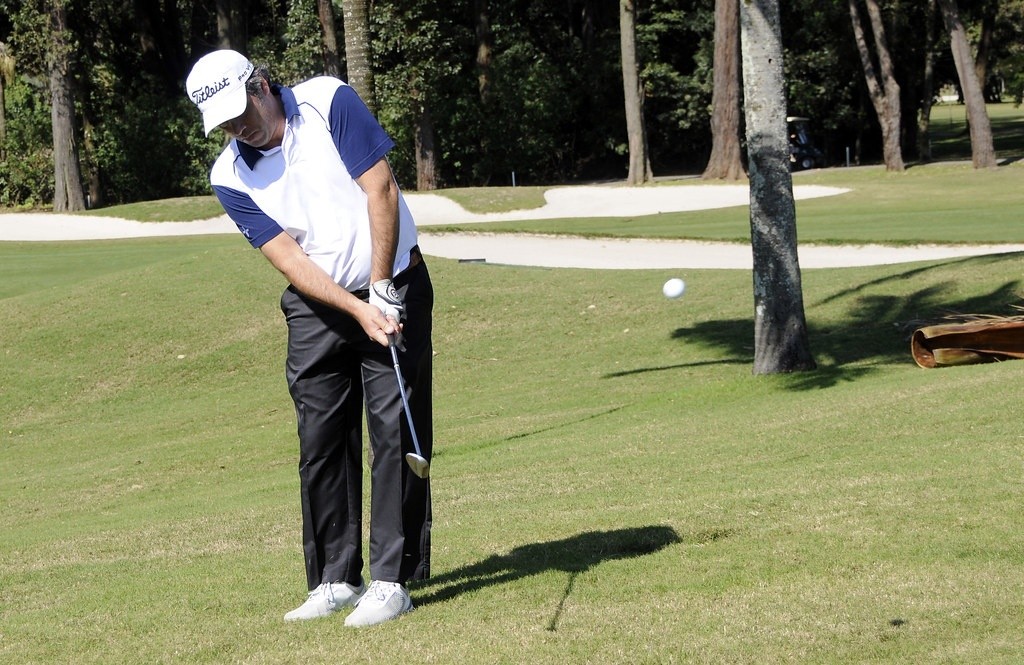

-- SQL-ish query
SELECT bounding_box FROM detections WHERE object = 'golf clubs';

[385,331,429,479]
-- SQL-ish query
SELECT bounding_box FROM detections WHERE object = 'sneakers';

[346,578,413,627]
[284,579,365,620]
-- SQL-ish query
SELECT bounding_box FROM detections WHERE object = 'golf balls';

[662,278,685,298]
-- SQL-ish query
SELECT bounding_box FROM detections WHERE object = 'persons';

[186,50,433,627]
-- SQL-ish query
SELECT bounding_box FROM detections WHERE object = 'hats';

[187,48,256,137]
